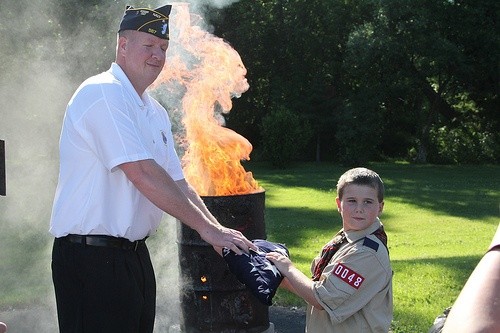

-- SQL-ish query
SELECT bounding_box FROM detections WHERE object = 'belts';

[58,234,145,253]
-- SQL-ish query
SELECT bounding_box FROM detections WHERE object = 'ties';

[311,225,389,281]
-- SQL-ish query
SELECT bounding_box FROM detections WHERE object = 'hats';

[117,5,172,40]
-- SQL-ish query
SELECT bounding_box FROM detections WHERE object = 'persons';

[264,168,394,333]
[442,222,500,333]
[50,4,258,333]
